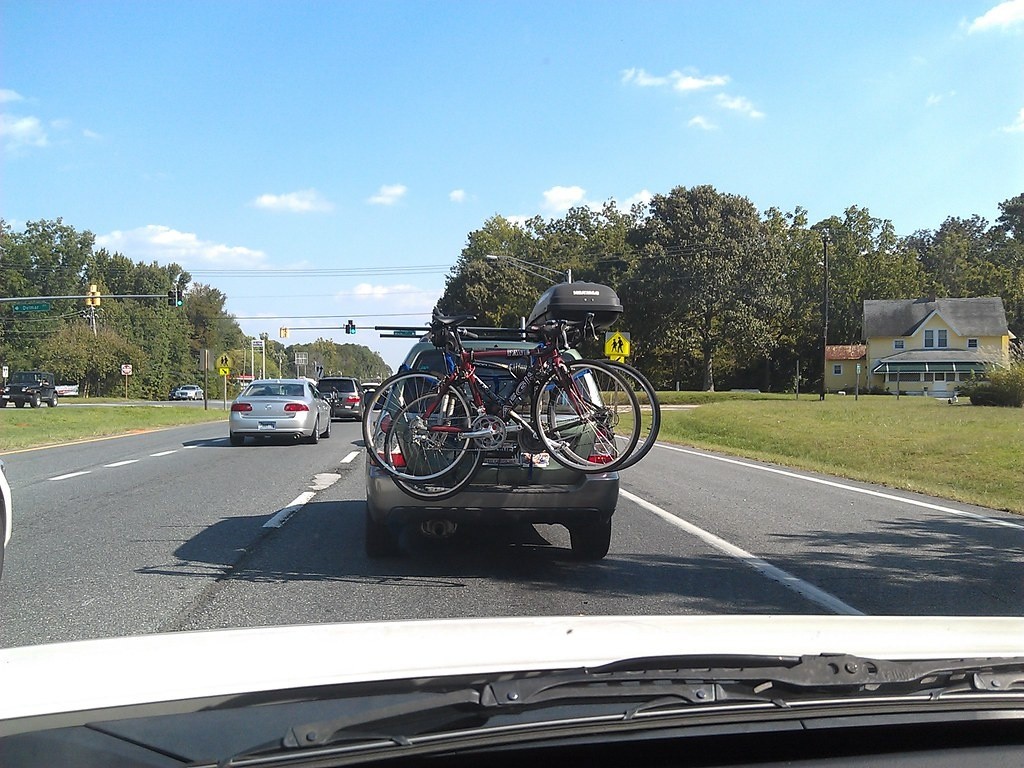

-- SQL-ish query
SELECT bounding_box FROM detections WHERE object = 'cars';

[168,385,204,400]
[360,382,387,411]
[230,379,333,447]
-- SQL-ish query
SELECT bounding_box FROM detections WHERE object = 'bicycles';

[359,309,663,502]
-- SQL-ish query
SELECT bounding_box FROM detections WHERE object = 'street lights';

[486,255,572,284]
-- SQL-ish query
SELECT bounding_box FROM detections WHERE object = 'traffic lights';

[177,290,183,306]
[351,325,355,334]
[346,325,350,334]
[347,320,353,324]
[169,290,176,305]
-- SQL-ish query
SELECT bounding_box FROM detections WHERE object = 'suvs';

[316,377,366,420]
[368,326,620,562]
[0,371,58,409]
